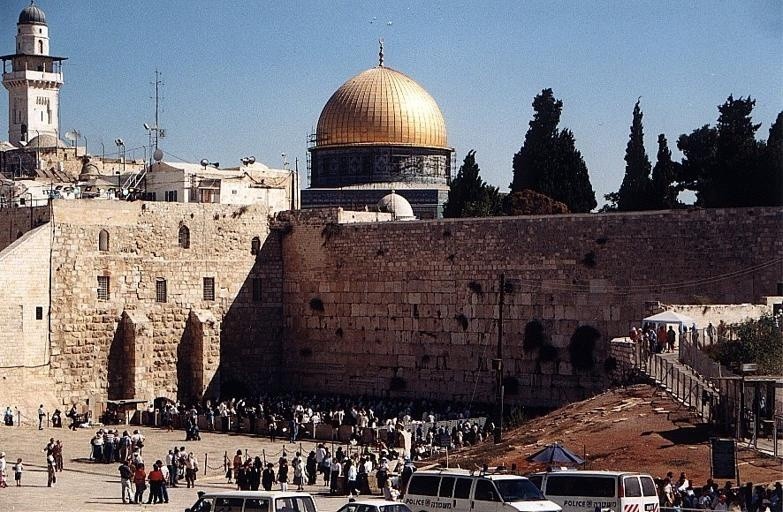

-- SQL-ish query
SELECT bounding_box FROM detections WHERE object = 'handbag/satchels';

[52,471,57,484]
[193,463,199,472]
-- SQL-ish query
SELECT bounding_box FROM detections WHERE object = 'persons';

[651,472,782,512]
[751,392,767,431]
[629,317,729,364]
[1,390,496,512]
[33,215,42,227]
[15,226,23,239]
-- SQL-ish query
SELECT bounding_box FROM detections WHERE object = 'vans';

[185,489,317,511]
[527,470,661,511]
[403,468,563,512]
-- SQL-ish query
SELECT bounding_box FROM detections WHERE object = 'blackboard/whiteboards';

[710,438,737,480]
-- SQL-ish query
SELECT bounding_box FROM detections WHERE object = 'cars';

[334,500,413,511]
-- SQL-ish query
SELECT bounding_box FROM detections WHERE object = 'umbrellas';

[522,441,586,470]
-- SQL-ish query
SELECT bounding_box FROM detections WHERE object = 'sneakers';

[296,487,304,490]
[345,495,354,498]
[156,501,170,504]
[143,500,156,504]
[122,500,133,504]
[355,490,361,498]
[134,501,143,504]
[186,484,195,488]
